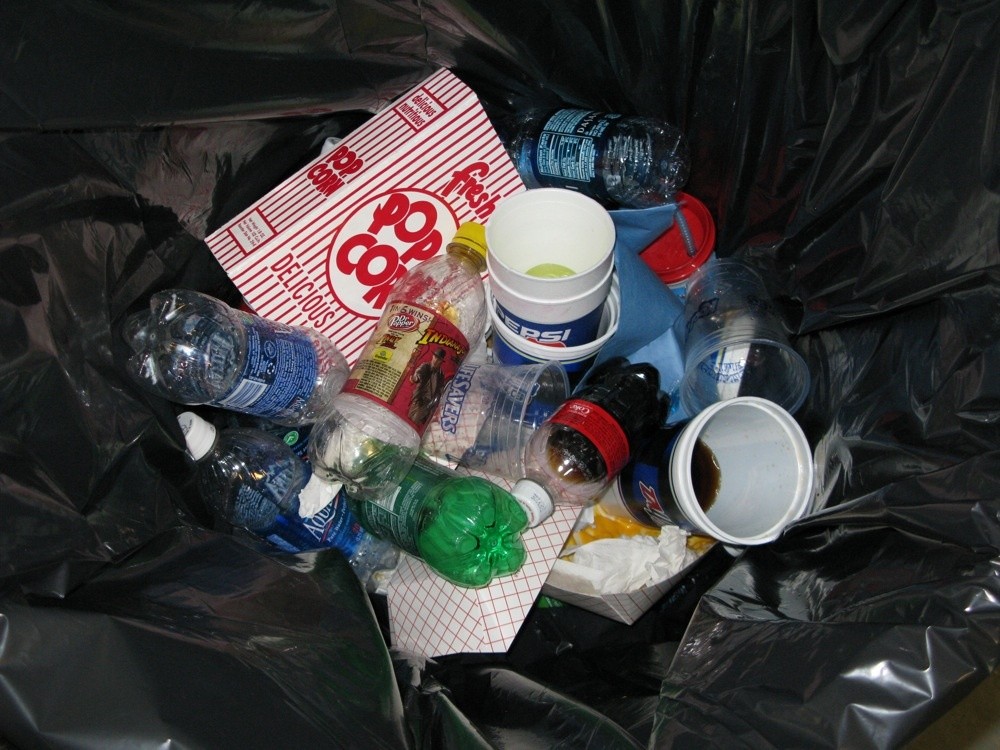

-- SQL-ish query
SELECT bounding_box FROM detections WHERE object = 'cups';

[598,396,815,546]
[420,359,571,481]
[678,258,813,418]
[484,188,623,428]
[634,190,718,306]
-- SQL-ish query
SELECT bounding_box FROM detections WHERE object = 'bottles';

[497,102,692,211]
[350,453,529,589]
[307,221,488,503]
[509,355,672,529]
[176,411,401,594]
[118,287,350,428]
